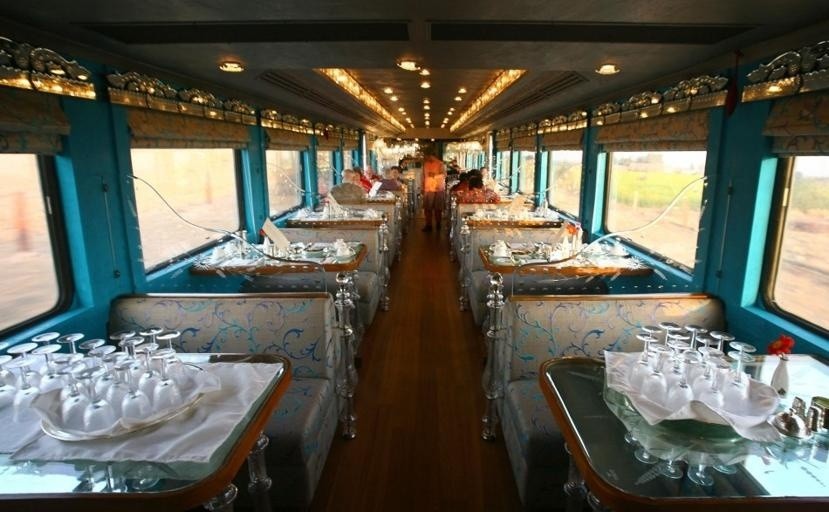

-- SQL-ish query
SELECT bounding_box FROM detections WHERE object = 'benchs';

[107,177,414,507]
[452,191,727,505]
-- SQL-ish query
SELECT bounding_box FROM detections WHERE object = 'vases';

[770,357,790,394]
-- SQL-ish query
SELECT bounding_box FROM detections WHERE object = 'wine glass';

[0,327,198,434]
[624,431,739,487]
[76,461,161,494]
[629,320,758,415]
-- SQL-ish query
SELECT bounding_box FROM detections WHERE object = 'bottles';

[262,236,271,260]
[770,355,790,400]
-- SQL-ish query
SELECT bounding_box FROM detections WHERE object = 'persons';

[341,159,408,194]
[449,159,500,203]
[415,147,447,233]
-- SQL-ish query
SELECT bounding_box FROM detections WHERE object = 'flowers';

[768,335,796,359]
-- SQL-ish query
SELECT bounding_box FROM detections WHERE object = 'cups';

[334,238,350,257]
[489,239,507,257]
